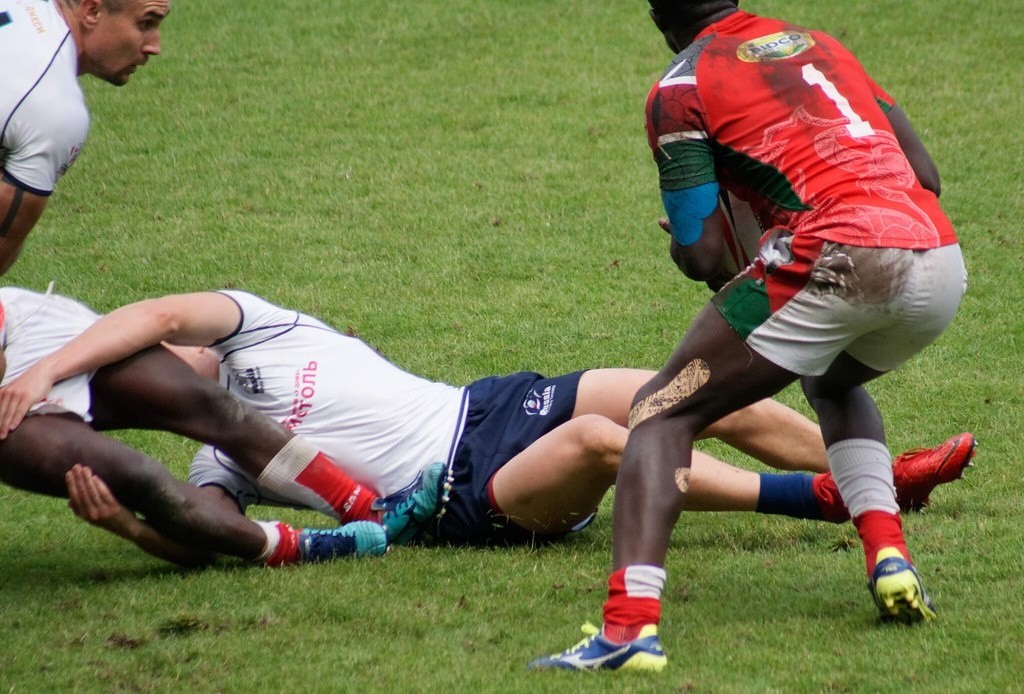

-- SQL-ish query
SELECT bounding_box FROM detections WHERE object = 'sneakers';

[894,434,978,512]
[527,621,668,671]
[868,546,938,623]
[298,520,389,564]
[814,473,852,524]
[371,462,454,546]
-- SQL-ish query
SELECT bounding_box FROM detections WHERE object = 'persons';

[0,0,171,276]
[533,0,967,672]
[1,288,978,567]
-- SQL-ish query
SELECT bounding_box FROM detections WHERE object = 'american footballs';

[712,187,767,276]
